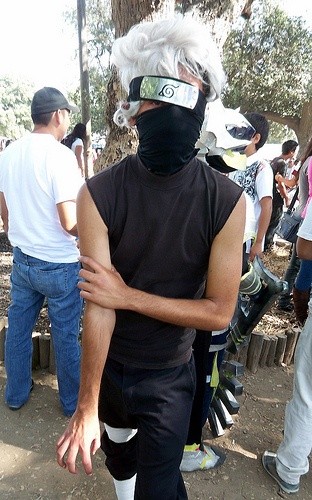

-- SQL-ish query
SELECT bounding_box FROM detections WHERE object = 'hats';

[31,86,78,117]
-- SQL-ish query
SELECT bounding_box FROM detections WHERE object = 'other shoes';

[9,379,34,410]
[278,304,294,310]
[180,444,226,472]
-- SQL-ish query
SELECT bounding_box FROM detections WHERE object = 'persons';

[0,91,312,494]
[54,16,247,500]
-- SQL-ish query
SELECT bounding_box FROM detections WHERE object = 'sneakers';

[262,449,300,493]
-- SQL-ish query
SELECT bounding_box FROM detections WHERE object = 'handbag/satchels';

[276,213,300,243]
